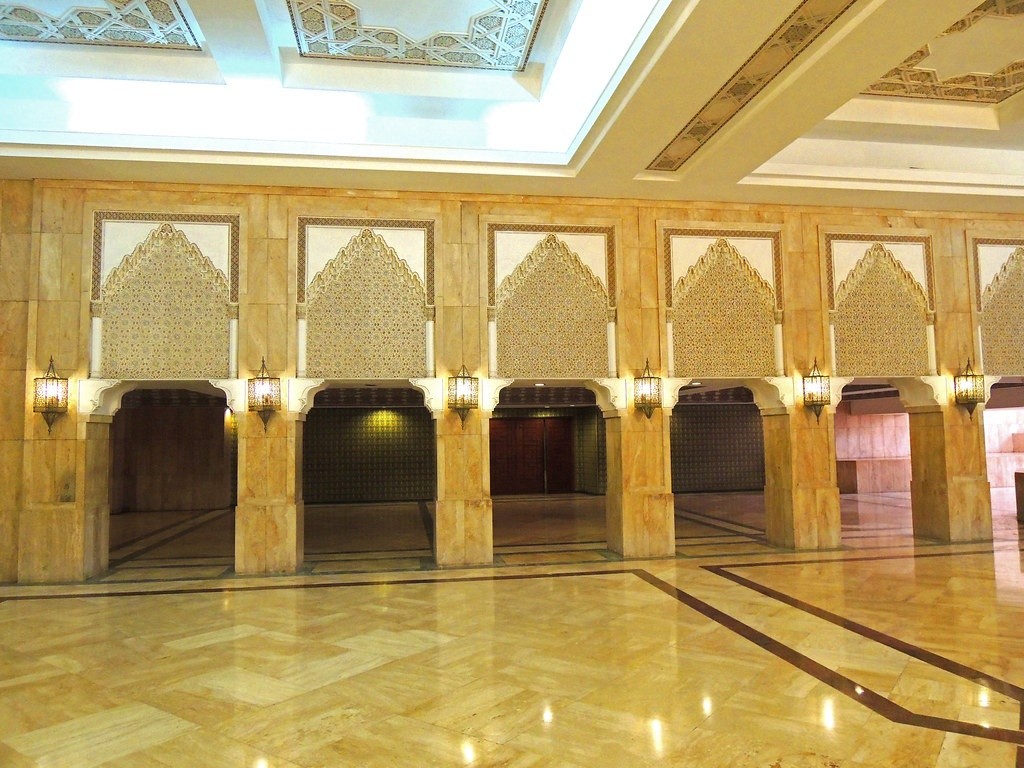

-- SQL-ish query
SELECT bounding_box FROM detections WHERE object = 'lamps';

[448,365,478,430]
[954,357,985,421]
[248,355,281,433]
[634,358,663,419]
[803,357,830,425]
[33,355,68,436]
